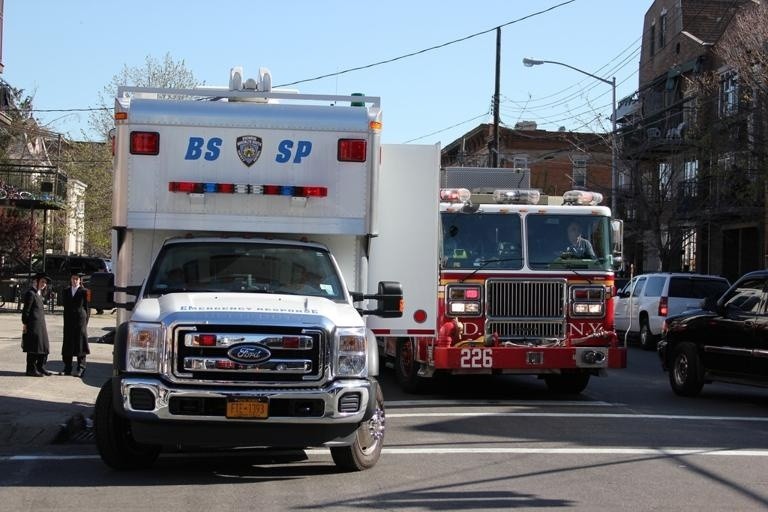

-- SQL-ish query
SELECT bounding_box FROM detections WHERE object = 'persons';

[277,263,321,295]
[59,274,92,378]
[443,225,459,270]
[553,221,604,269]
[21,272,53,376]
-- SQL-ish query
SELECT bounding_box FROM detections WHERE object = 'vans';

[615,272,733,347]
[0,253,112,304]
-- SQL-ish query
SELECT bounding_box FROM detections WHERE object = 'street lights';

[523,55,618,218]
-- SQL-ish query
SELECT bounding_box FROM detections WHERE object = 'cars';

[655,268,767,396]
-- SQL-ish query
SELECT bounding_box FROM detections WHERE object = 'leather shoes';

[59,366,72,375]
[26,369,42,376]
[37,367,49,375]
[74,366,85,377]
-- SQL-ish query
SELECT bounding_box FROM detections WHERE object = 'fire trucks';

[379,167,625,392]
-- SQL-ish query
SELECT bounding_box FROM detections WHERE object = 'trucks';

[92,67,402,471]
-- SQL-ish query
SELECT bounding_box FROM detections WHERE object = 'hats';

[32,271,52,285]
[71,269,86,277]
